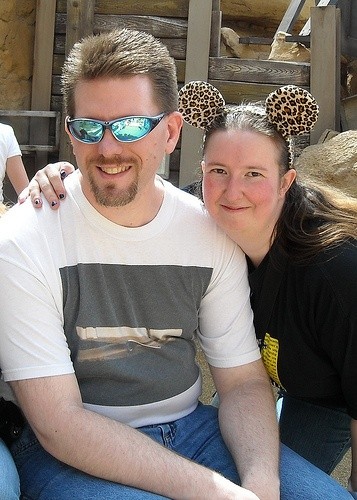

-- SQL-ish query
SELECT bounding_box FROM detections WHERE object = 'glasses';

[65,109,171,145]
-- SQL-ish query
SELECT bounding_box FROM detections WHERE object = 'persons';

[0,123,31,214]
[0,436,22,500]
[0,29,355,500]
[17,109,357,495]
